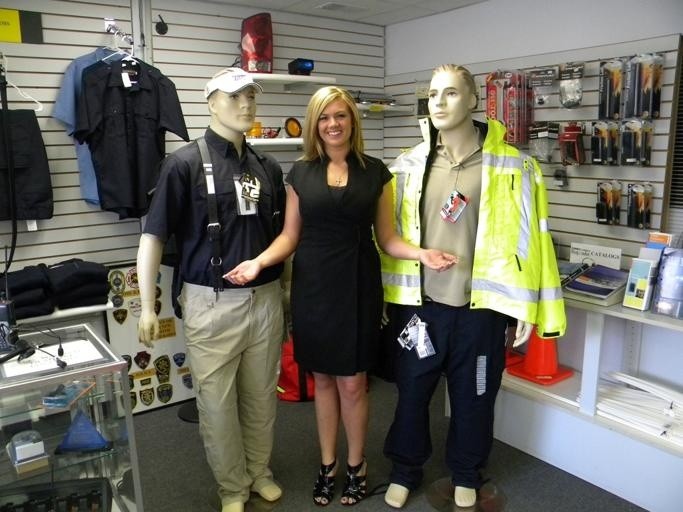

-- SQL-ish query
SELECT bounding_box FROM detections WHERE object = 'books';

[557,259,629,306]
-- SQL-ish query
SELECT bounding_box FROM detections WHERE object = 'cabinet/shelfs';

[245,73,416,146]
[1,323,142,512]
[445,260,683,511]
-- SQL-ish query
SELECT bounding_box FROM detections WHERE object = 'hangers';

[99,26,142,82]
[0,58,45,113]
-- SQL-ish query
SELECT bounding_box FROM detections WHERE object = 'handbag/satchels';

[277,338,369,401]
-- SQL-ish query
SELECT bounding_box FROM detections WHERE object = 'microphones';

[14,321,64,357]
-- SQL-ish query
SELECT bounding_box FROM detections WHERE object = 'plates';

[285,117,302,137]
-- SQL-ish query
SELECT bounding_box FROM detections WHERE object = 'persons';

[222,86,457,505]
[137,68,286,511]
[385,63,566,508]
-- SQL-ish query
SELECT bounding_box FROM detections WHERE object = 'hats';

[204,67,264,99]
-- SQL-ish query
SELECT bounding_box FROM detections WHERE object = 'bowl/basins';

[261,127,281,138]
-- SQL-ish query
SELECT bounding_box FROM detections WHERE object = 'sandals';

[342,460,367,506]
[313,457,336,507]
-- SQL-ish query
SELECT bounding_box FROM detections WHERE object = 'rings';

[241,283,245,285]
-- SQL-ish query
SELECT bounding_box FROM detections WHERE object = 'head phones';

[0,324,33,365]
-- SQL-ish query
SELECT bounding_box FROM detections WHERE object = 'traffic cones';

[506,316,575,388]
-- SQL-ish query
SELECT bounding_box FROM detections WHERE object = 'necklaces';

[327,167,347,184]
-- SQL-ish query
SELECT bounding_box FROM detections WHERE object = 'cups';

[246,122,261,138]
